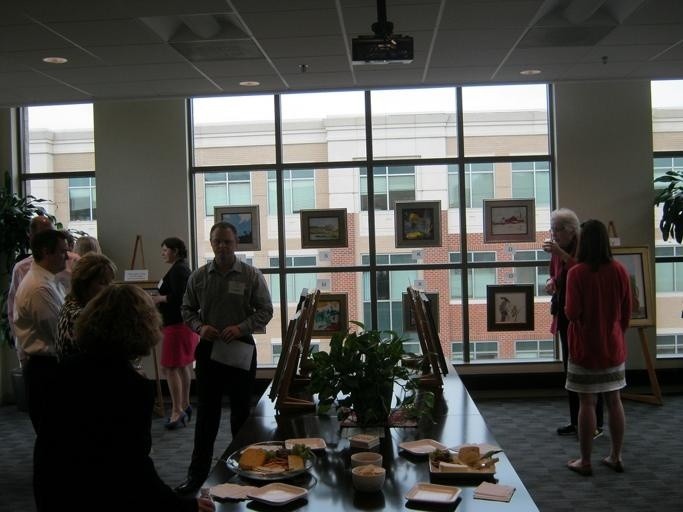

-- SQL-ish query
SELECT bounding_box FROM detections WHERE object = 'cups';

[543,238,553,252]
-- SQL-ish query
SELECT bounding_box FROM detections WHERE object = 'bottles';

[199,488,213,502]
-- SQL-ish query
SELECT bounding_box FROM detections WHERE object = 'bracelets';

[197,324,203,334]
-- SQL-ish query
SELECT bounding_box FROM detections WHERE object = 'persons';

[173,222,273,497]
[31,283,216,512]
[543,207,604,444]
[12,216,148,434]
[564,218,633,476]
[149,236,199,429]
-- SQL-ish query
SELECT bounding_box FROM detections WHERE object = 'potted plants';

[301,321,438,431]
[0,172,89,412]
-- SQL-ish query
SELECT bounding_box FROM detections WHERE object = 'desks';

[252,357,481,414]
[195,414,538,511]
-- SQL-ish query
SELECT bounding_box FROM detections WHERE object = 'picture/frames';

[402,291,440,333]
[304,293,350,338]
[214,204,261,252]
[394,200,442,249]
[483,198,537,244]
[299,208,349,250]
[610,246,655,326]
[486,283,535,332]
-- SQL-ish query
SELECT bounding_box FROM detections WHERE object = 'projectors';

[351,34,415,65]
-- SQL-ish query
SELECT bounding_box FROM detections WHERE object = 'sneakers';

[599,454,624,473]
[592,427,604,439]
[567,458,592,476]
[557,424,578,436]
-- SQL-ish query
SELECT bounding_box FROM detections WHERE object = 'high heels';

[164,406,192,429]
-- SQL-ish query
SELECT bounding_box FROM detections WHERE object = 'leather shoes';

[176,480,196,494]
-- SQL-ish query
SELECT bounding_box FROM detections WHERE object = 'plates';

[284,437,327,451]
[428,451,501,474]
[404,481,463,504]
[397,438,450,457]
[245,481,309,507]
[224,440,318,481]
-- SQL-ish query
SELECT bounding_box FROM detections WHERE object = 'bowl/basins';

[350,451,384,469]
[351,465,387,493]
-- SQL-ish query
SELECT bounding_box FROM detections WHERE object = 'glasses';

[551,227,564,232]
